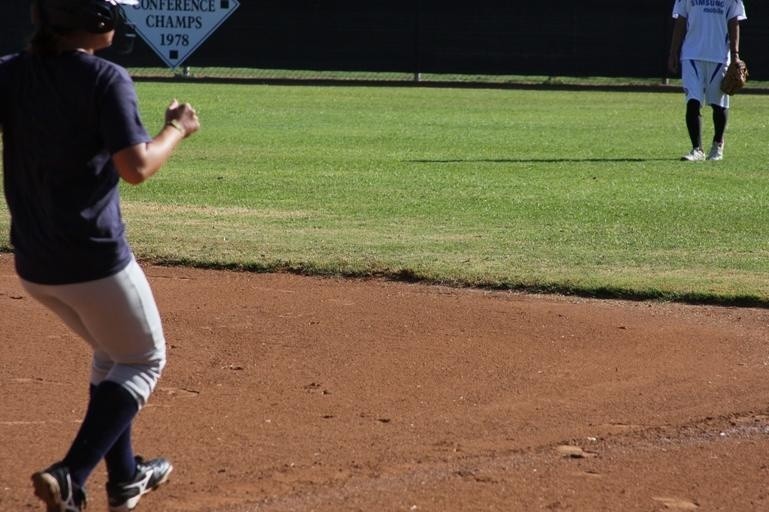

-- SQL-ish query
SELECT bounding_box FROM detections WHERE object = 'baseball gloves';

[720,59,748,96]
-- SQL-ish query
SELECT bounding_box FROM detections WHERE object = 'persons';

[665,1,750,162]
[1,0,204,512]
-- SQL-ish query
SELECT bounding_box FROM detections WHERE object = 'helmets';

[29,0,120,30]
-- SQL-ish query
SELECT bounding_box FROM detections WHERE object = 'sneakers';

[681,147,706,161]
[708,135,724,161]
[106,455,173,512]
[32,462,87,512]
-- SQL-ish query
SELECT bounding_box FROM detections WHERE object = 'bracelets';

[165,117,186,136]
